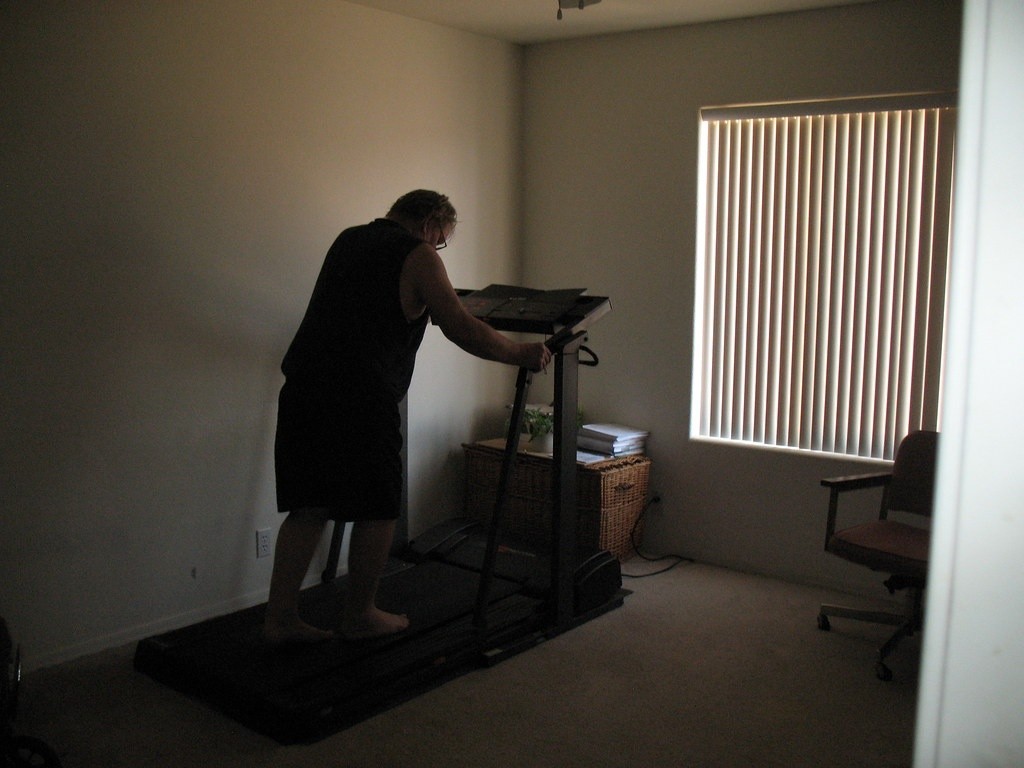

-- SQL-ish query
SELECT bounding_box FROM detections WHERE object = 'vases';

[530,431,554,452]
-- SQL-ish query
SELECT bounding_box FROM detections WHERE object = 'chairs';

[817,430,938,682]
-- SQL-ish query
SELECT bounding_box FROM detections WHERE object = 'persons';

[261,190,552,648]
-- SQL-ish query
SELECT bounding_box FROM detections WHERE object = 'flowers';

[503,397,586,443]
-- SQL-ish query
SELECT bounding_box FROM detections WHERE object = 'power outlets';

[255,528,272,558]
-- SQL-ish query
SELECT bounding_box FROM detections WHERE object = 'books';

[576,422,652,456]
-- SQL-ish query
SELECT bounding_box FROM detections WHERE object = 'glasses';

[435,221,447,250]
